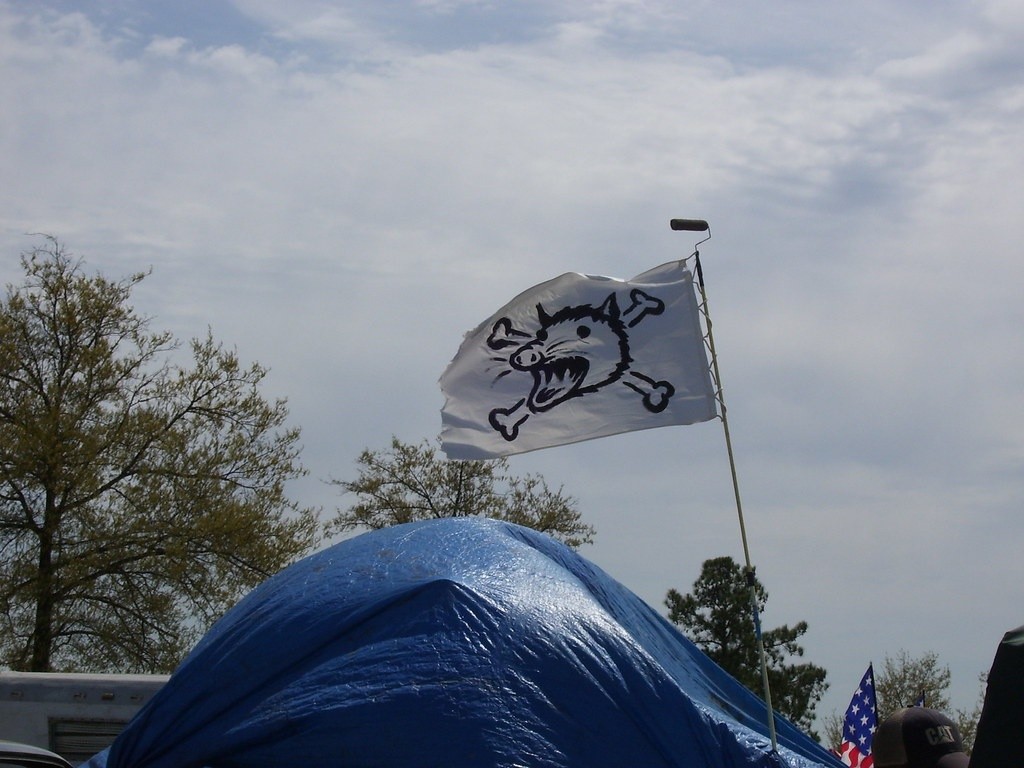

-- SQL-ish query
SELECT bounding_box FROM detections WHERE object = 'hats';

[871,704,964,768]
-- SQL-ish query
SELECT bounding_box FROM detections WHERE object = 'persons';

[871,705,970,768]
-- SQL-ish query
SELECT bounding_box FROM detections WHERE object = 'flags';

[839,662,878,768]
[436,257,718,462]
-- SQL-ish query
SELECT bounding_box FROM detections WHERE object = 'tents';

[80,516,848,768]
